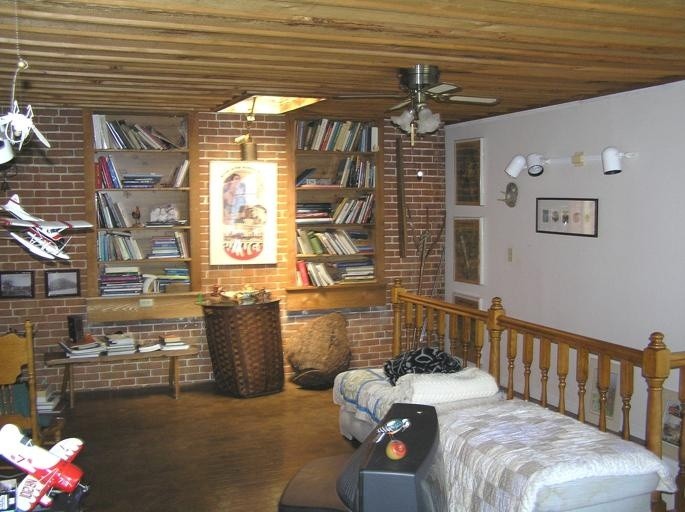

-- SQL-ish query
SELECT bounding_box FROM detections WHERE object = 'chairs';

[0,319,64,470]
[277,405,383,511]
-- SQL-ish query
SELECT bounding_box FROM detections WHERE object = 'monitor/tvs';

[277,402,449,512]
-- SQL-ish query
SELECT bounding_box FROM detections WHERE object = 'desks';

[196,297,285,398]
[16,397,69,414]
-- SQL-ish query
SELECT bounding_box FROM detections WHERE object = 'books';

[90,105,196,297]
[292,115,381,291]
[53,312,192,359]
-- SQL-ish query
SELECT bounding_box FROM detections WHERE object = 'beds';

[331,352,680,511]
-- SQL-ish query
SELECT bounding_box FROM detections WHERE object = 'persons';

[223,172,262,226]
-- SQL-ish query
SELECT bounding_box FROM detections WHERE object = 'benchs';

[43,343,199,408]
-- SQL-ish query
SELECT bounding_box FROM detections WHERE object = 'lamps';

[246,96,258,128]
[504,145,638,179]
[391,106,447,147]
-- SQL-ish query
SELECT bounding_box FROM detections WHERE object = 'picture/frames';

[582,354,625,434]
[660,384,683,461]
[535,195,601,239]
[452,215,485,288]
[449,292,483,366]
[453,136,486,206]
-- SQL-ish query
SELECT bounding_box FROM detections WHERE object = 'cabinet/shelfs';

[284,110,386,311]
[80,107,204,323]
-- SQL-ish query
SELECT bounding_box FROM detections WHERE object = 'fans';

[333,62,499,116]
